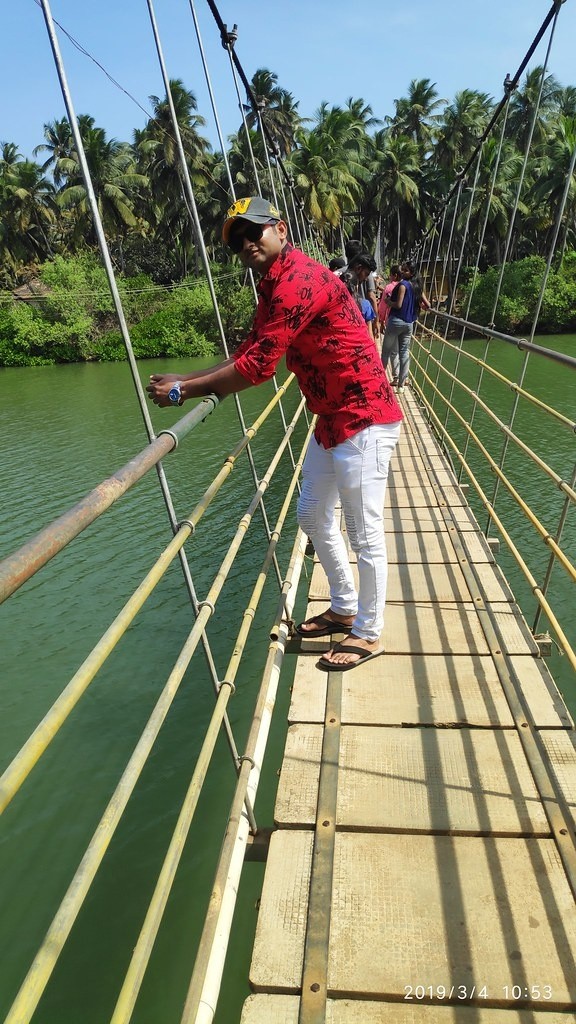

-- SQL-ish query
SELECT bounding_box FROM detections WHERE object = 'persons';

[327,240,431,393]
[146,198,405,672]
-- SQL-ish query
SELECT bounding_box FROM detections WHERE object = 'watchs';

[168,381,184,407]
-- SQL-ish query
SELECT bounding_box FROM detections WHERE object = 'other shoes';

[404,380,409,386]
[389,381,398,386]
[397,387,405,394]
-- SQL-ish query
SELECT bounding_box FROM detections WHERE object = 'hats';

[221,196,281,241]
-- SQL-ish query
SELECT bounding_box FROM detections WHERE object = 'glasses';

[228,223,266,251]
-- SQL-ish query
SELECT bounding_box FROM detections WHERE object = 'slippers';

[295,613,353,638]
[319,641,386,671]
